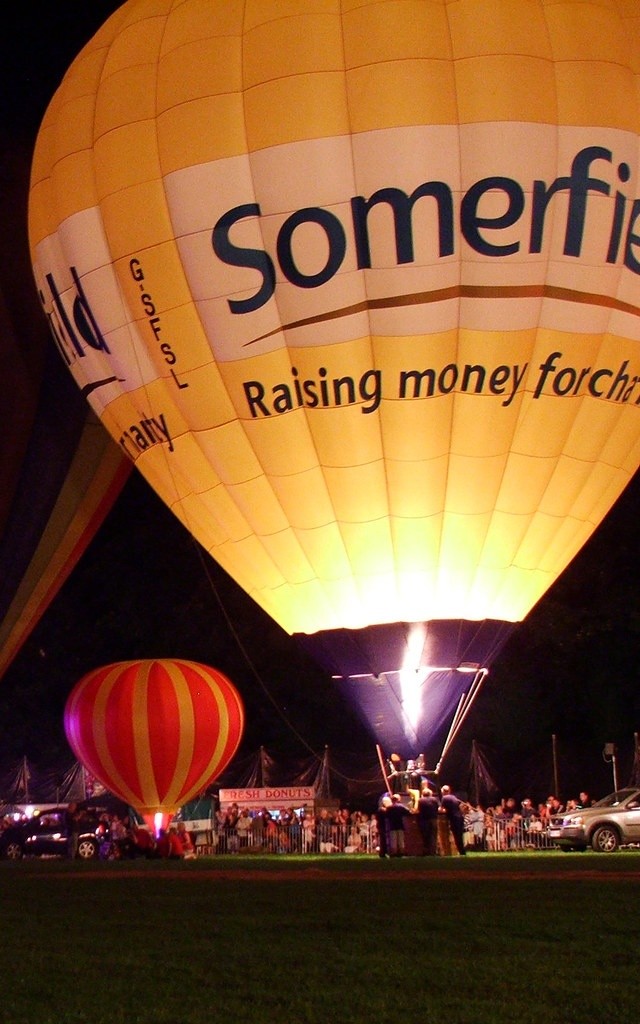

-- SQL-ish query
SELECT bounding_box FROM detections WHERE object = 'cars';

[0,806,106,860]
[547,788,640,853]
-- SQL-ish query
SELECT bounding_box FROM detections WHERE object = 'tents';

[218,786,316,816]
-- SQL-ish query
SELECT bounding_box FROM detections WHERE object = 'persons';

[64,801,87,863]
[381,758,466,857]
[213,801,380,854]
[93,813,198,861]
[458,792,597,851]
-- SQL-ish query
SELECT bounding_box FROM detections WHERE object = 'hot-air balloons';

[63,658,244,843]
[27,0,640,857]
